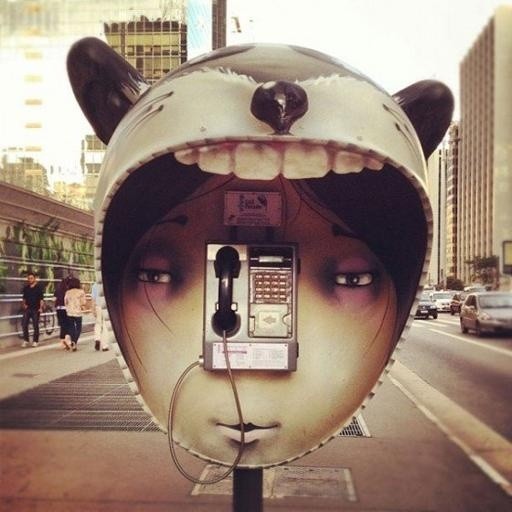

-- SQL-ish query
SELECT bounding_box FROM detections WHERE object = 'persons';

[91,283,110,352]
[64,277,87,351]
[53,277,71,351]
[20,271,44,348]
[104,150,430,469]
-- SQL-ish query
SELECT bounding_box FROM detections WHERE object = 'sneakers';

[63,340,80,352]
[21,341,40,348]
[95,340,108,351]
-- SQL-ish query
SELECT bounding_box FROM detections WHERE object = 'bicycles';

[14,301,55,339]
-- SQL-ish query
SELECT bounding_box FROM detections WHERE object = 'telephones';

[203,242,297,371]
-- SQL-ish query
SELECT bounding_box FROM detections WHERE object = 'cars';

[414,282,511,338]
